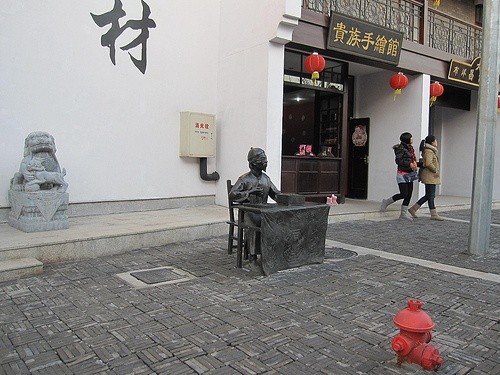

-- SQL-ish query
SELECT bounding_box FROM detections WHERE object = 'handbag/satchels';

[403,171,418,183]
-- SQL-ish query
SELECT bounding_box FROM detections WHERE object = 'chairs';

[226,180,249,259]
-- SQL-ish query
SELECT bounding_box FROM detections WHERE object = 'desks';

[234,203,330,274]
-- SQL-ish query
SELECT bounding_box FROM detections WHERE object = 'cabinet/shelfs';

[318,109,338,156]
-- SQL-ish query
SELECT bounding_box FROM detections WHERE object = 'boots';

[430,209,444,221]
[399,205,413,222]
[379,197,395,212]
[408,202,421,218]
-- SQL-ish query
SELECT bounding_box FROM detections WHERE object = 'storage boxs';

[278,195,306,206]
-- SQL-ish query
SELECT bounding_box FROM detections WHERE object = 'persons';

[229,147,280,273]
[379,132,425,220]
[408,136,445,221]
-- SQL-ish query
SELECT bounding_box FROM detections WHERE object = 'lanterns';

[429,80,444,107]
[497,93,500,111]
[305,52,325,80]
[389,71,407,101]
